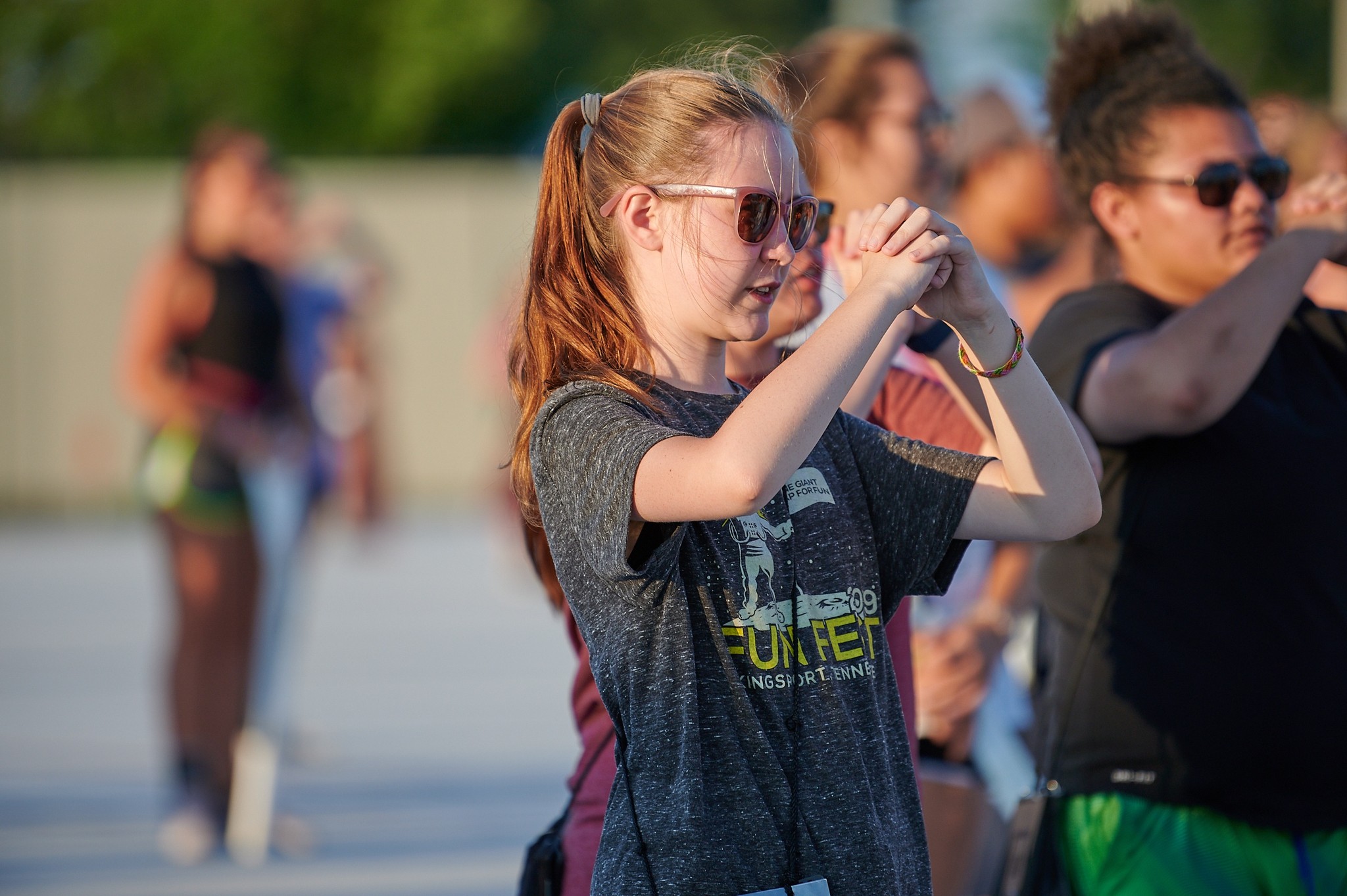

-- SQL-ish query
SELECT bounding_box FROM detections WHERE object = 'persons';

[497,5,1347,896]
[118,120,395,868]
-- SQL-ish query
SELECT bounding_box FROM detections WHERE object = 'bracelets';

[957,318,1024,378]
[905,320,952,354]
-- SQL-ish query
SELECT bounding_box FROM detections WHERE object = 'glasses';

[1113,152,1292,209]
[600,184,819,253]
[819,202,834,243]
[845,97,953,137]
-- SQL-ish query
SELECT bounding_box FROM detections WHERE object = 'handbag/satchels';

[995,795,1064,896]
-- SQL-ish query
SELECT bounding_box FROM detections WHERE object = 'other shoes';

[157,803,213,868]
